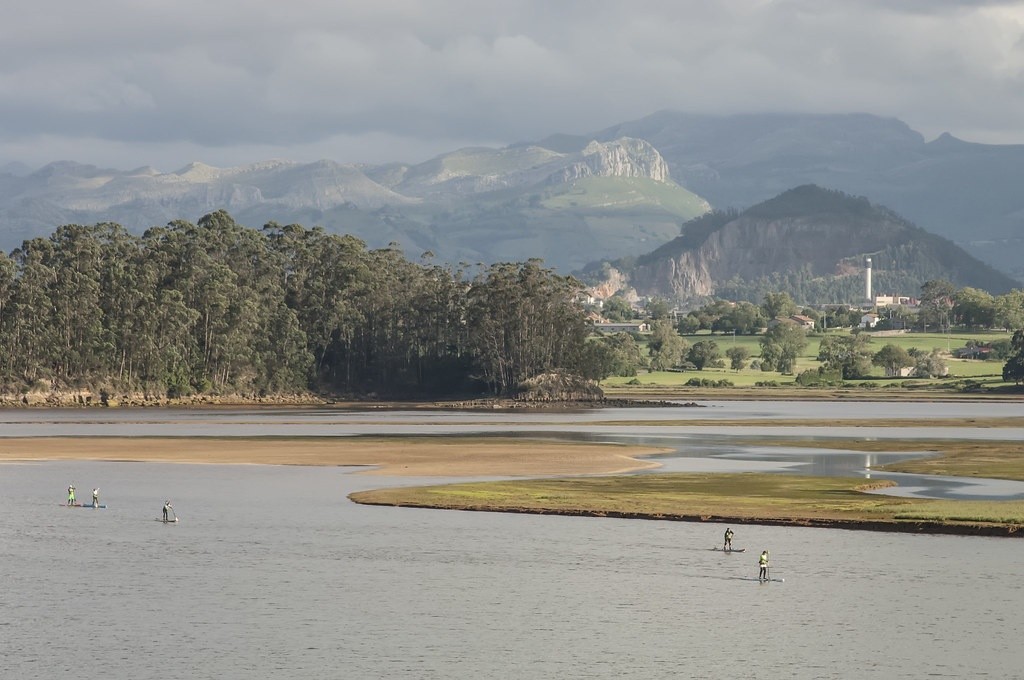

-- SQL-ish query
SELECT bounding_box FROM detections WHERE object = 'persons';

[759,551,768,579]
[92,488,100,505]
[163,501,170,521]
[68,485,75,504]
[724,528,734,550]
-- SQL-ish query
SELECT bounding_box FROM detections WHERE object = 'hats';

[69,485,72,487]
